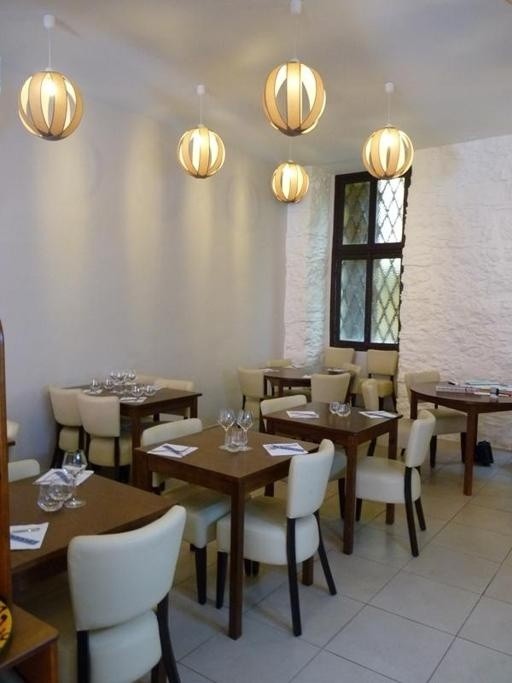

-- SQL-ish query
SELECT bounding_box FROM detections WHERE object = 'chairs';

[48,385,89,471]
[352,409,436,556]
[16,505,187,683]
[260,395,349,522]
[215,439,337,637]
[140,418,253,605]
[362,379,427,458]
[238,367,277,428]
[406,370,470,469]
[352,350,398,411]
[80,391,138,481]
[139,379,201,428]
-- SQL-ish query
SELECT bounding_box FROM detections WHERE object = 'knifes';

[366,412,394,421]
[152,448,173,452]
[12,527,39,533]
[291,412,316,417]
[273,445,304,453]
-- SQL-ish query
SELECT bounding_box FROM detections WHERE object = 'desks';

[257,366,355,398]
[410,380,512,497]
[62,383,204,448]
[10,466,184,683]
[134,423,324,642]
[258,401,404,555]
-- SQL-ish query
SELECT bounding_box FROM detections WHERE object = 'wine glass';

[217,408,254,451]
[102,370,136,397]
[64,450,87,508]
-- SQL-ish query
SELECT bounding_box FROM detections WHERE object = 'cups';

[133,385,155,396]
[38,478,74,513]
[327,401,351,416]
[224,429,248,450]
[88,376,102,389]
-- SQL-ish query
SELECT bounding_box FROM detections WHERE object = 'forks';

[164,444,189,454]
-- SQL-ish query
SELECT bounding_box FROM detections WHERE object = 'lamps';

[178,82,227,181]
[17,14,84,144]
[264,1,328,136]
[363,82,416,180]
[271,134,311,205]
